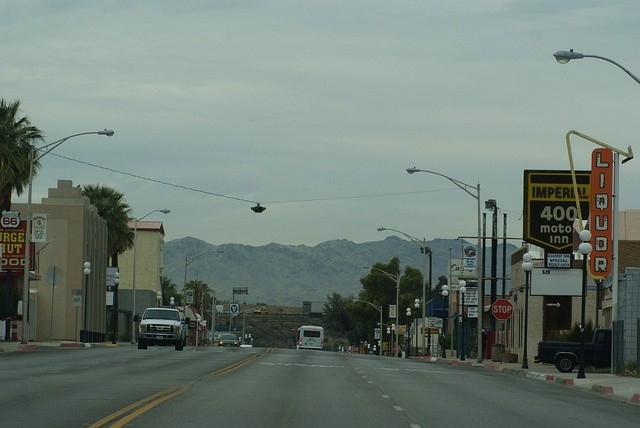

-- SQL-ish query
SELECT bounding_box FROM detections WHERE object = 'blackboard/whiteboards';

[254,308,270,315]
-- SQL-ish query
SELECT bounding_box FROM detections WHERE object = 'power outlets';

[534,328,612,373]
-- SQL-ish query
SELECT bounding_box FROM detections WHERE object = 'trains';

[491,299,513,320]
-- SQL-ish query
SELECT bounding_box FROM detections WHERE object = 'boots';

[133,306,191,351]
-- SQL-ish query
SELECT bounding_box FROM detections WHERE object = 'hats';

[220,334,239,347]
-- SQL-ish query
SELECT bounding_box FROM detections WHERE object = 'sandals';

[297,325,324,350]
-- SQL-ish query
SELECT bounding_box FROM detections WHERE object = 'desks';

[157,291,162,308]
[352,298,384,357]
[552,48,640,85]
[169,296,175,308]
[441,285,449,358]
[391,324,395,357]
[130,208,171,345]
[83,261,91,343]
[576,229,592,378]
[376,225,426,357]
[406,307,412,359]
[414,299,420,357]
[111,272,120,344]
[362,264,401,358]
[521,253,533,369]
[17,128,115,343]
[182,250,225,347]
[459,280,466,361]
[406,164,483,363]
[386,327,391,356]
